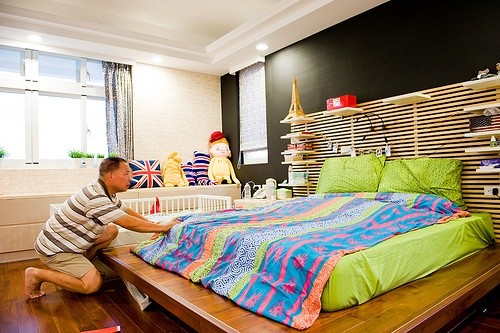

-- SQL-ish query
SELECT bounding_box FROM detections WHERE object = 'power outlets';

[484,186,500,197]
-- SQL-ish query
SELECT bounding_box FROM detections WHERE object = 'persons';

[24,158,183,298]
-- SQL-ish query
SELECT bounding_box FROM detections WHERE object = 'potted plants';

[84,151,95,167]
[96,153,103,166]
[67,148,83,167]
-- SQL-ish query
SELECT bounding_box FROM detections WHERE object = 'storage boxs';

[326,94,357,111]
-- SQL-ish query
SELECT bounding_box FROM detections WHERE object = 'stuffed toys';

[207,131,240,185]
[162,152,189,187]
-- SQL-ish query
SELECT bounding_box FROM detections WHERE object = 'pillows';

[378,158,467,211]
[127,160,162,188]
[180,151,217,185]
[313,152,385,194]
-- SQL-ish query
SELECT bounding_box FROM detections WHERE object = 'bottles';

[244,183,251,199]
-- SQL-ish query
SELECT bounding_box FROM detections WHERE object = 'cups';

[276,189,292,200]
[266,178,276,200]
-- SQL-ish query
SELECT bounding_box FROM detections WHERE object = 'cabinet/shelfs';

[462,76,500,174]
[277,116,315,187]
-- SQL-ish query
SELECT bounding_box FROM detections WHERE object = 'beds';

[97,81,500,333]
